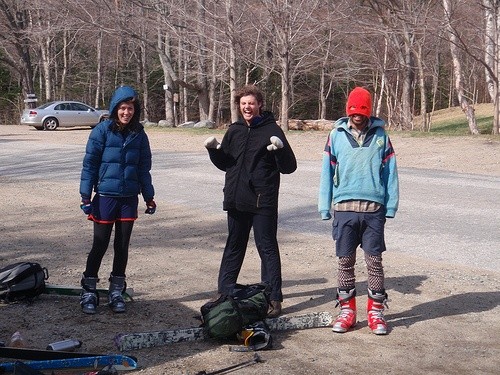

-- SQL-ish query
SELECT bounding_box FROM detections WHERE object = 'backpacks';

[193,282,274,340]
[0,262,49,303]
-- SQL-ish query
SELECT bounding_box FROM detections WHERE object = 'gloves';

[267,136,284,151]
[145,200,156,214]
[237,320,273,349]
[204,136,221,149]
[80,196,92,214]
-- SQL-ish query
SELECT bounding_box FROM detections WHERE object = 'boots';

[332,288,357,332]
[367,288,388,334]
[80,275,98,314]
[107,274,126,312]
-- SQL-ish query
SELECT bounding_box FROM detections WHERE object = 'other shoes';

[267,301,281,318]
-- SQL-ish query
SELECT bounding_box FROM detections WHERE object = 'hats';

[346,87,372,118]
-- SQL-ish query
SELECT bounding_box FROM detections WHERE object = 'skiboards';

[45,284,134,303]
[115,312,333,350]
[0,346,138,371]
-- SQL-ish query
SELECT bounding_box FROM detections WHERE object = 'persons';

[204,85,297,317]
[317,87,399,335]
[79,86,157,314]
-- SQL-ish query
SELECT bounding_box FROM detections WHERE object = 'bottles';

[11,332,25,348]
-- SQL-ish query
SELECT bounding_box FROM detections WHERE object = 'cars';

[20,101,110,131]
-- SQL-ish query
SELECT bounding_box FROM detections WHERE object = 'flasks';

[46,338,83,351]
